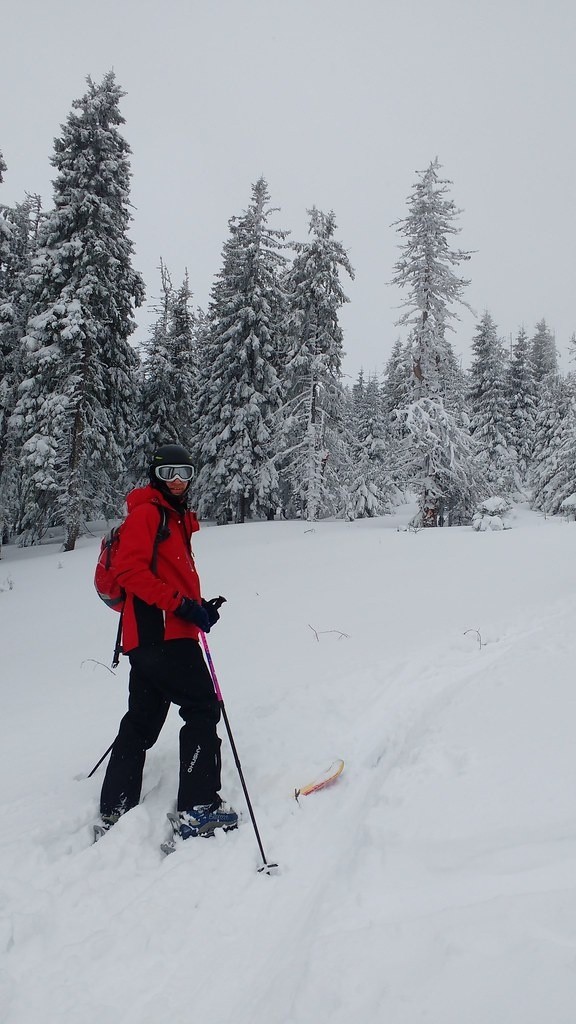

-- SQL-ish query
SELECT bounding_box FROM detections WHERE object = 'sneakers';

[178,802,238,835]
[102,812,120,825]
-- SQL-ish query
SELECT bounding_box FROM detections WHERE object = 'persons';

[100,444,237,841]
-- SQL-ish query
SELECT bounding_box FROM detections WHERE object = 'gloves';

[174,597,219,633]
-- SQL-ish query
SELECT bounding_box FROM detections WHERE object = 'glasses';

[154,464,195,482]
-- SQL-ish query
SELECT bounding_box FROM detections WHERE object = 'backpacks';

[94,502,166,613]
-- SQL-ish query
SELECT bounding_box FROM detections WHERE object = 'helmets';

[154,444,192,465]
